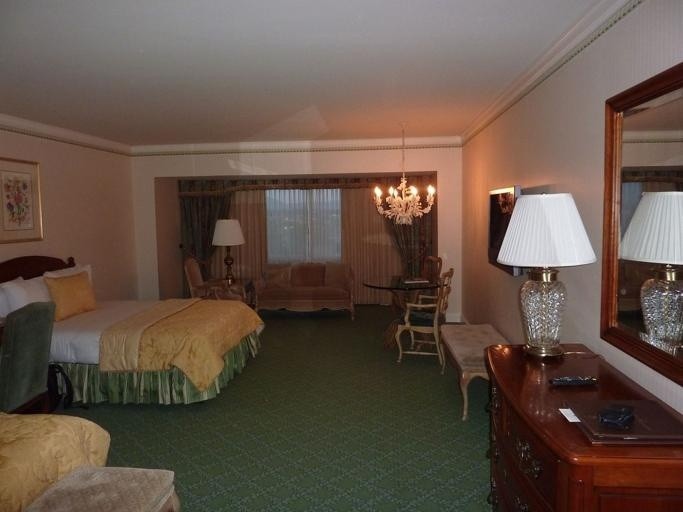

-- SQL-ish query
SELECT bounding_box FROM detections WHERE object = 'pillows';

[42,271,99,323]
[0,276,24,318]
[2,275,52,313]
[42,264,93,285]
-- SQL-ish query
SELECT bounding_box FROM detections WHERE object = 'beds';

[0,254,265,407]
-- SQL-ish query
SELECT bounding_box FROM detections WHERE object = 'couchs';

[254,261,355,321]
[613,259,654,312]
[0,410,110,511]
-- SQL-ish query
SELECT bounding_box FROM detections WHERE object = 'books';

[567,407,682,447]
[563,393,682,441]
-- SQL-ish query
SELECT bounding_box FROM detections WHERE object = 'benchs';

[21,466,182,512]
[437,322,512,420]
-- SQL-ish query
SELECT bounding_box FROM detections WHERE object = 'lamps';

[617,190,683,345]
[371,121,435,225]
[496,192,513,217]
[210,218,247,285]
[495,192,598,357]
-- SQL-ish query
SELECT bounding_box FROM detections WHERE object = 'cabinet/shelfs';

[482,342,681,512]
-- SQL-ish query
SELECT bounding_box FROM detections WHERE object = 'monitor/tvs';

[487,184,523,277]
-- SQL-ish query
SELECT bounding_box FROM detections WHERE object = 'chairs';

[1,300,57,414]
[418,255,442,279]
[393,267,453,365]
[181,256,243,301]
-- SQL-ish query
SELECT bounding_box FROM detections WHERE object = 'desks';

[362,276,447,350]
[212,278,256,310]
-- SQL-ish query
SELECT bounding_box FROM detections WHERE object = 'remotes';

[597,404,635,429]
[548,376,599,388]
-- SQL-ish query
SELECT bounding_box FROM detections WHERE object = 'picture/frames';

[0,155,46,244]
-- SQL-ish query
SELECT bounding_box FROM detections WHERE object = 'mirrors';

[598,59,682,387]
